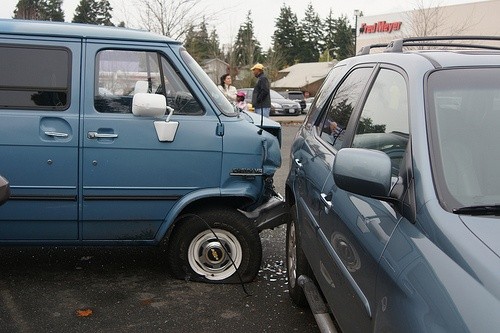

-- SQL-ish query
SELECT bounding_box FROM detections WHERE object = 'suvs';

[284,36,500,333]
[278,91,307,112]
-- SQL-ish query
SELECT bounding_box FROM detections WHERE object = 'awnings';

[270,73,326,88]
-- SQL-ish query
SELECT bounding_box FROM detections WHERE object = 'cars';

[237,88,302,116]
[351,130,411,178]
[302,97,315,114]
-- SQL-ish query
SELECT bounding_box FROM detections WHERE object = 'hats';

[250,64,264,70]
[236,92,245,96]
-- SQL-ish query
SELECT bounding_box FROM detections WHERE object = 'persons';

[217,74,237,103]
[323,122,337,135]
[235,92,247,110]
[250,63,271,117]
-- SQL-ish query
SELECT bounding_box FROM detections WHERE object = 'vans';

[0,18,288,285]
